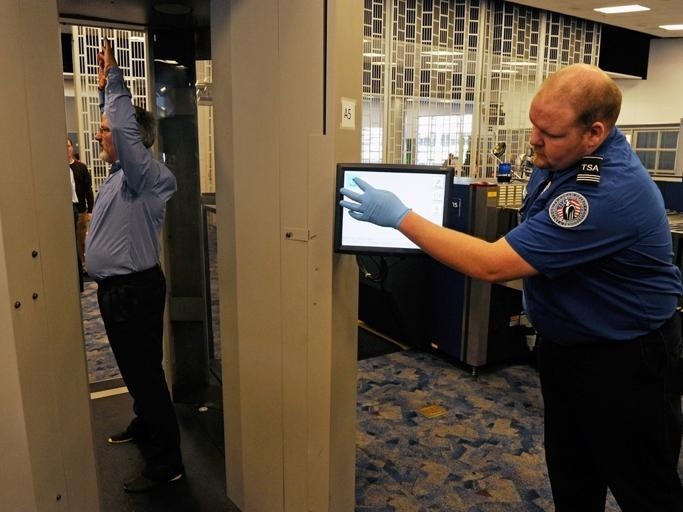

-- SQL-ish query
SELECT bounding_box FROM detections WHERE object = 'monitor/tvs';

[331,162,454,257]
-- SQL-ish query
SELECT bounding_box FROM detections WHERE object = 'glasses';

[99,128,109,133]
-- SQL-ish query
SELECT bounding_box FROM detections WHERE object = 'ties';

[521,170,554,223]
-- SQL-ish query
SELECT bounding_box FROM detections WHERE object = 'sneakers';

[107,430,137,443]
[123,464,184,493]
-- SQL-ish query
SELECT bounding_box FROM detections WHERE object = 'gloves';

[339,177,412,229]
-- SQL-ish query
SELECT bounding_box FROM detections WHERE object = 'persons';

[66,137,94,294]
[84,36,185,492]
[444,153,453,165]
[339,61,683,512]
